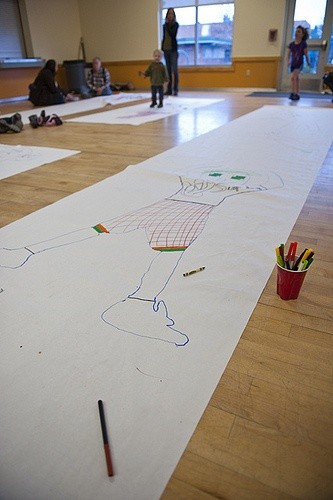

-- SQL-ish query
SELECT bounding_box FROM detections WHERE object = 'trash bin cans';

[63,59,87,91]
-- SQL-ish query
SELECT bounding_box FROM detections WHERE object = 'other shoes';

[173,92,178,96]
[289,93,299,100]
[164,90,172,95]
[150,101,157,107]
[158,102,163,108]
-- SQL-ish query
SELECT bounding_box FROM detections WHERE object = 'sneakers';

[0,113,23,134]
[29,110,45,128]
[45,113,62,127]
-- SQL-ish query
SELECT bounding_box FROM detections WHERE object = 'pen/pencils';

[183,267,206,277]
[98,399,115,477]
[275,240,314,271]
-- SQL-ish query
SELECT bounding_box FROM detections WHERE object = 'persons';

[286,25,310,100]
[28,59,79,106]
[143,49,169,108]
[79,57,112,99]
[161,7,180,95]
